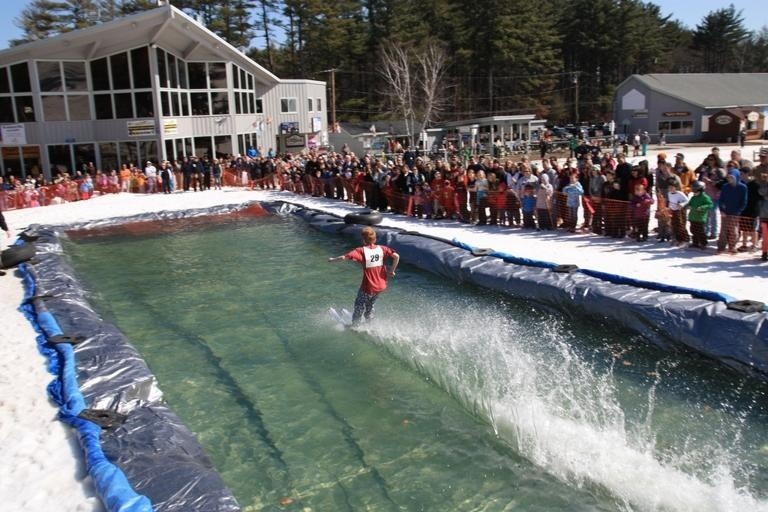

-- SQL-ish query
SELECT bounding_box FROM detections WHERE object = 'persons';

[641,132,650,156]
[448,142,454,153]
[740,131,746,147]
[341,143,351,155]
[632,134,640,156]
[539,139,546,159]
[0,148,285,211]
[329,226,401,328]
[387,138,402,154]
[623,136,629,155]
[654,147,766,258]
[570,136,579,159]
[611,139,619,159]
[286,143,653,242]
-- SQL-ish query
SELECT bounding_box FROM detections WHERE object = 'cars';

[553,123,610,139]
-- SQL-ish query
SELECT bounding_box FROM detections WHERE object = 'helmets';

[692,181,706,190]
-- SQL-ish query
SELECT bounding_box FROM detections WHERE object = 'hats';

[674,153,684,158]
[758,148,768,157]
